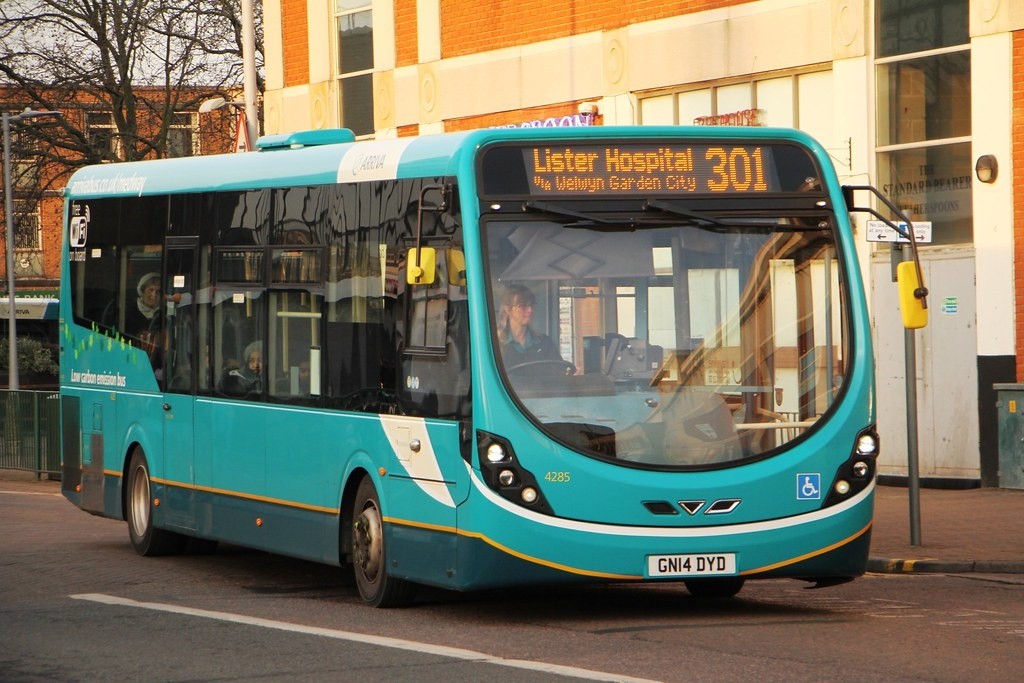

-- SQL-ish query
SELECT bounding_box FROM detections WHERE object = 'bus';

[59,124,932,614]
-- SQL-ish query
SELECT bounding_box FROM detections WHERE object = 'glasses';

[509,301,537,309]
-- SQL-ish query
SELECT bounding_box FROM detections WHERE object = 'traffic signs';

[866,220,932,245]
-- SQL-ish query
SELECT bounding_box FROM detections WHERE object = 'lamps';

[975,154,998,184]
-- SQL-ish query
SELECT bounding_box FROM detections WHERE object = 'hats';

[137,272,162,298]
[243,340,263,367]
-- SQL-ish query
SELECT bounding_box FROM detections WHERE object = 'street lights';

[197,101,260,153]
[2,107,65,457]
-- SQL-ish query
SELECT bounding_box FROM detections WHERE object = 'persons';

[497,284,578,378]
[124,271,311,396]
